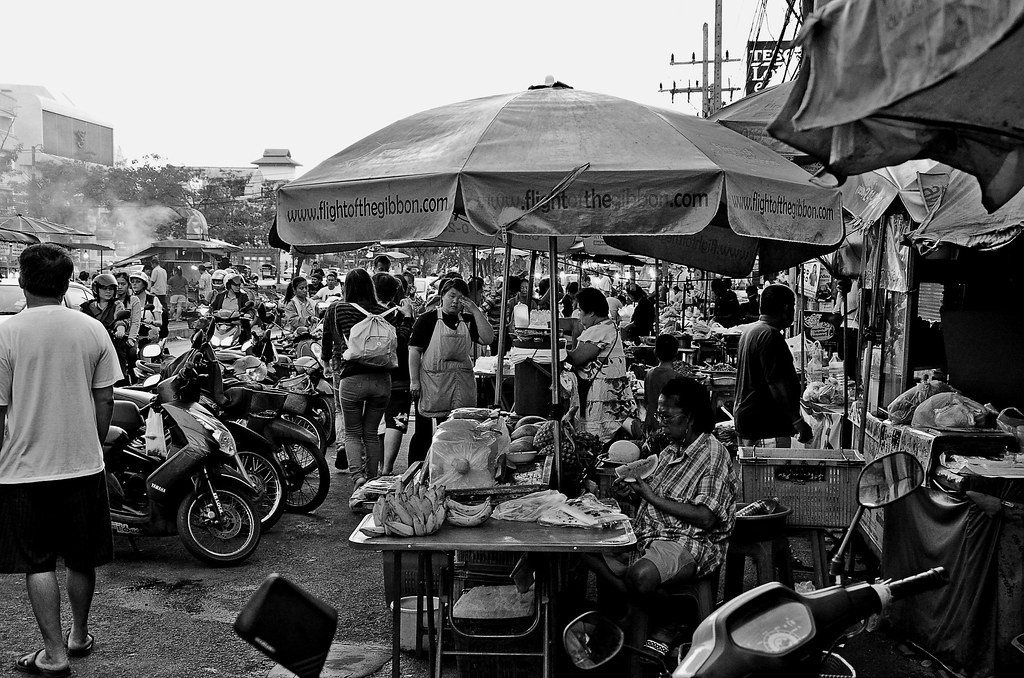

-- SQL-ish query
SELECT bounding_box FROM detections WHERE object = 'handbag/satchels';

[141,408,168,460]
[806,339,822,364]
[828,313,842,330]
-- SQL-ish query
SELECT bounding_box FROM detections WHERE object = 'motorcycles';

[102,293,341,567]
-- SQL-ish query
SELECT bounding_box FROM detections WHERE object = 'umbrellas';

[0,213,95,246]
[269,76,847,592]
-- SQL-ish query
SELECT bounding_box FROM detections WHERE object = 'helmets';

[224,273,241,292]
[294,356,319,376]
[250,273,258,282]
[129,272,148,287]
[92,274,118,298]
[211,270,228,289]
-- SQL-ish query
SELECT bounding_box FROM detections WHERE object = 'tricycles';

[262,264,277,280]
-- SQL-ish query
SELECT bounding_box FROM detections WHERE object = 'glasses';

[98,286,114,290]
[131,280,140,283]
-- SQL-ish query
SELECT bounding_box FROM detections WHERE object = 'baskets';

[250,389,288,420]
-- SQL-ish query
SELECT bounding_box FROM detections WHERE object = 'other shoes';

[335,444,348,469]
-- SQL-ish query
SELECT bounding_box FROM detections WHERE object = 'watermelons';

[615,454,659,482]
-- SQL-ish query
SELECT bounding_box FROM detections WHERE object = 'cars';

[329,267,341,277]
[340,269,348,275]
[283,268,308,281]
[411,268,419,274]
[0,279,95,325]
[322,268,329,277]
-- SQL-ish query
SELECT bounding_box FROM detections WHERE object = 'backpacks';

[343,303,399,368]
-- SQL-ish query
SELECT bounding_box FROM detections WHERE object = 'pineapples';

[532,419,600,463]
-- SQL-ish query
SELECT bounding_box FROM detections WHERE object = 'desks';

[346,505,635,678]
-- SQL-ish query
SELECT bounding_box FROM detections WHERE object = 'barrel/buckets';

[390,596,448,654]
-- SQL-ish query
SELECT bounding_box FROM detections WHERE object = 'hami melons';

[608,441,640,462]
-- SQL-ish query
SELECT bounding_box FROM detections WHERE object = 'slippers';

[16,648,70,678]
[65,627,94,657]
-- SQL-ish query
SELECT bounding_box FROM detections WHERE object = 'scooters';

[232,572,339,678]
[561,451,949,678]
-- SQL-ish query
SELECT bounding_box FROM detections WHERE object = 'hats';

[205,262,212,267]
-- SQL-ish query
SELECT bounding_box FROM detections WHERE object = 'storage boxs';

[737,445,866,528]
[383,550,525,610]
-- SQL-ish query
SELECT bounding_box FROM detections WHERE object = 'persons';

[150,259,167,308]
[733,285,813,448]
[578,378,736,664]
[282,261,344,334]
[321,255,654,477]
[78,270,163,388]
[711,278,759,329]
[168,266,188,322]
[644,334,686,431]
[0,244,125,678]
[195,262,257,345]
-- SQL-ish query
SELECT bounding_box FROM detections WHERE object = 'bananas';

[358,480,452,538]
[445,495,493,527]
